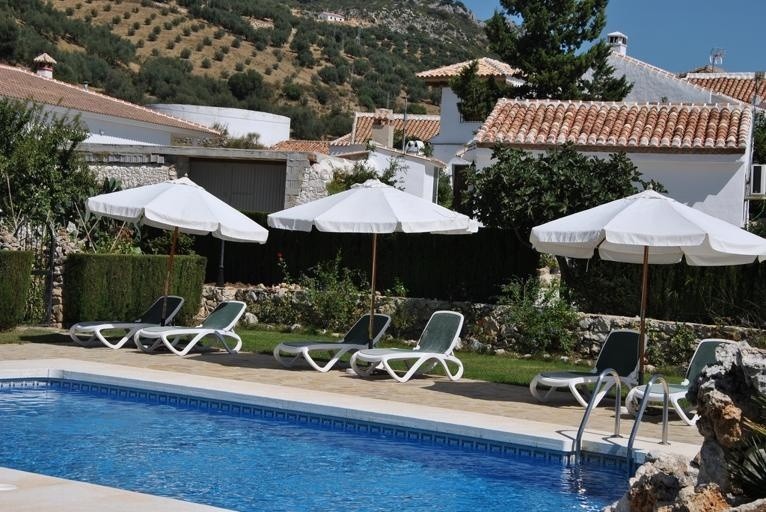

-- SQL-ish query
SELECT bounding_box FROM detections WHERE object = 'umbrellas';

[85,172,269,327]
[527,180,766,407]
[267,174,480,367]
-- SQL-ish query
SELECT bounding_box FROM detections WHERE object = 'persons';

[405,133,425,156]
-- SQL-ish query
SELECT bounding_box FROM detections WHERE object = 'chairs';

[273,313,392,372]
[350,310,464,382]
[134,300,248,356]
[624,338,738,426]
[69,294,185,349]
[529,329,648,410]
